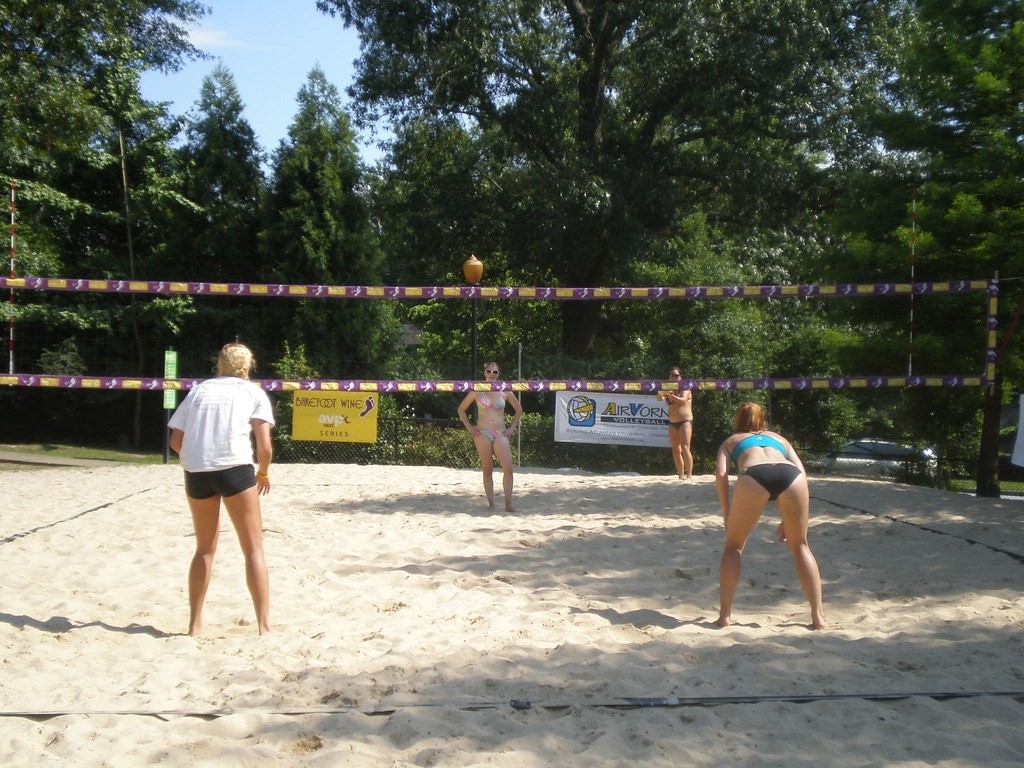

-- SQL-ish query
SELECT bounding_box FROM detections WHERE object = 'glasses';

[669,374,680,377]
[486,370,498,374]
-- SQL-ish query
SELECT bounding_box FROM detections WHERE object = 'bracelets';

[257,473,270,478]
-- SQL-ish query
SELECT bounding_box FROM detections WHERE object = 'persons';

[167,344,276,636]
[716,402,826,629]
[658,368,694,480]
[458,362,522,513]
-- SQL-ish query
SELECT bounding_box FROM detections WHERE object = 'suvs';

[815,439,938,482]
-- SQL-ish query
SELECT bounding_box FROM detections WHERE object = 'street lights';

[461,255,482,420]
